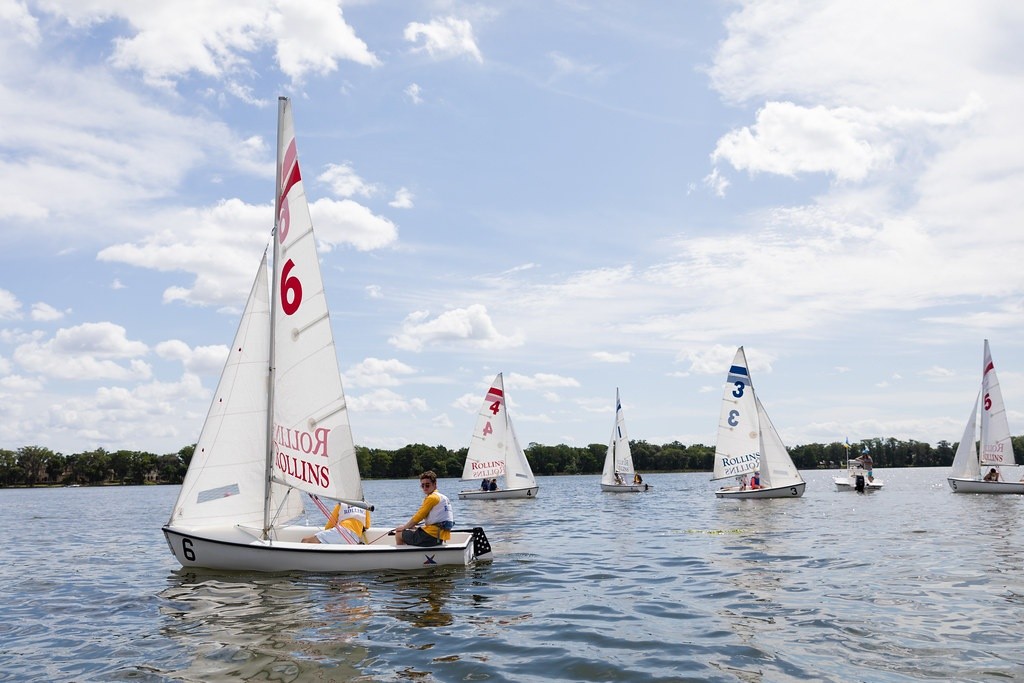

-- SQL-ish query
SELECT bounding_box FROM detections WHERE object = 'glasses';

[421,483,433,488]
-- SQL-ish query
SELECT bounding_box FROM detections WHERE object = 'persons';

[633,472,642,485]
[395,471,455,547]
[301,503,370,545]
[984,468,999,481]
[615,473,622,485]
[750,472,766,490]
[479,479,499,491]
[736,475,749,491]
[855,450,874,483]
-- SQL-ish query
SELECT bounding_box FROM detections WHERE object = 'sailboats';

[709,345,806,500]
[457,372,540,499]
[946,338,1024,495]
[162,94,477,571]
[599,387,649,493]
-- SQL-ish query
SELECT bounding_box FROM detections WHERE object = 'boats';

[835,437,883,492]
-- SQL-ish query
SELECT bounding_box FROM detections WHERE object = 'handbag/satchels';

[432,519,454,530]
